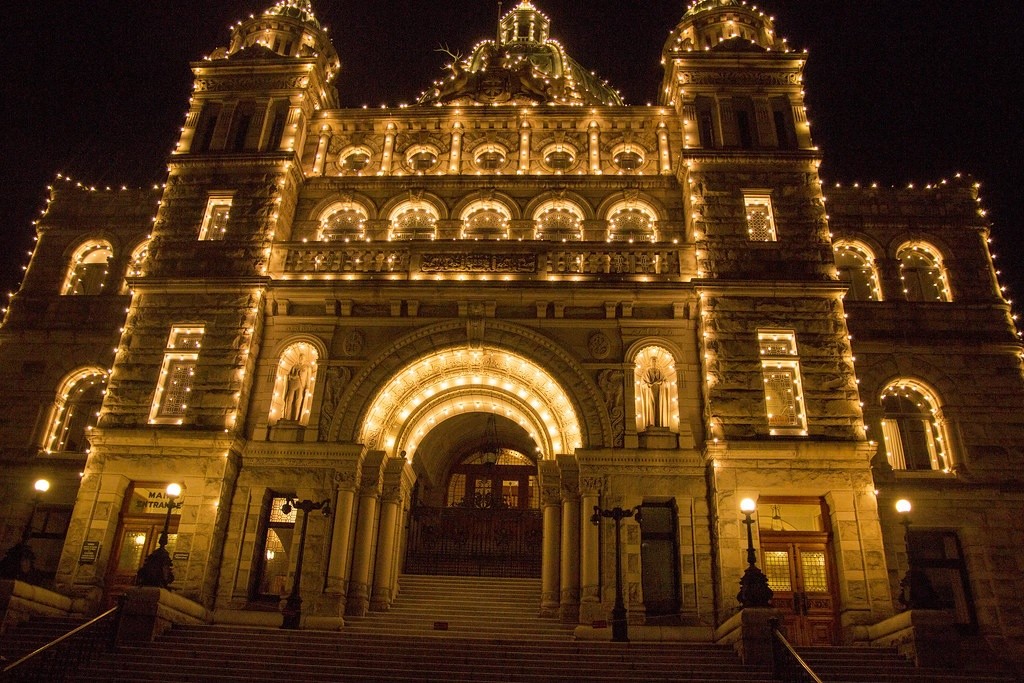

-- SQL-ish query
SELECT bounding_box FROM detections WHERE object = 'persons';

[640,355,671,431]
[283,351,311,422]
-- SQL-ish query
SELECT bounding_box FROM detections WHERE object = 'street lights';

[280,495,332,630]
[590,506,645,641]
